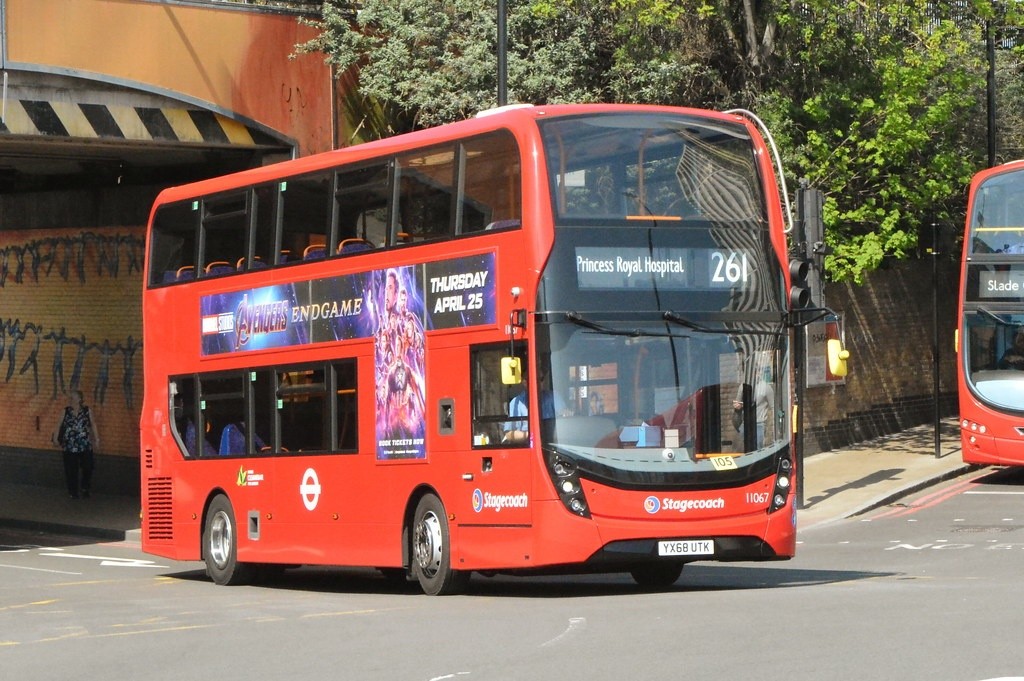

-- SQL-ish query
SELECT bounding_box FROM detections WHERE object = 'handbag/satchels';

[51,426,66,444]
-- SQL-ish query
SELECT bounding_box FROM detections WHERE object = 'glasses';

[524,376,544,382]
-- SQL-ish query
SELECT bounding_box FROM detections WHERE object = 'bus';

[952,158,1024,470]
[132,98,851,597]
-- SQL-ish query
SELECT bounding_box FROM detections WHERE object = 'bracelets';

[94,440,100,443]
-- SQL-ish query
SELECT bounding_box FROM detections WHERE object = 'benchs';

[159,232,413,287]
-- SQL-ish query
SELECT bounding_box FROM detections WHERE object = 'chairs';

[183,416,266,457]
[990,230,1024,252]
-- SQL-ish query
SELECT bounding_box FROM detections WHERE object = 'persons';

[52,390,101,501]
[371,269,424,414]
[497,365,570,444]
[997,326,1024,371]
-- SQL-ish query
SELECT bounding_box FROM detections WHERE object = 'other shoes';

[70,488,80,499]
[79,488,90,498]
